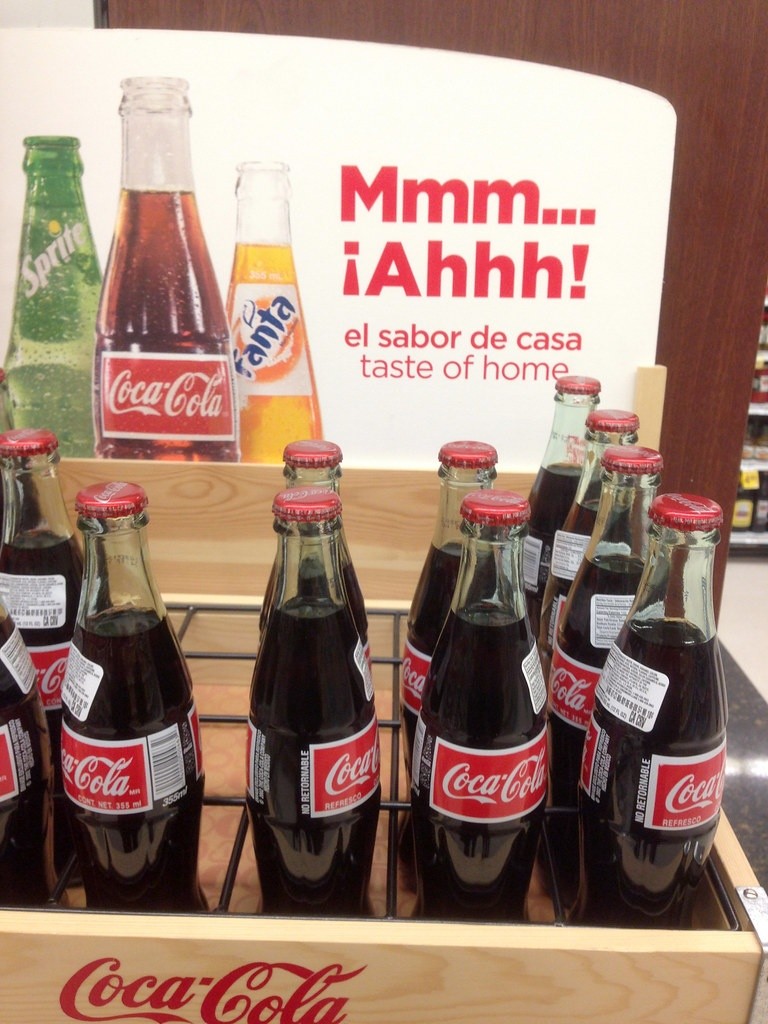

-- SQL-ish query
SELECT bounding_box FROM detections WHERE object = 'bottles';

[0,428,85,855]
[0,597,57,910]
[60,480,211,913]
[243,487,381,919]
[226,159,324,465]
[398,376,730,930]
[91,75,239,463]
[258,440,372,679]
[0,134,104,458]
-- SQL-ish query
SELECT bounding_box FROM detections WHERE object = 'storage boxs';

[0,456,768,1024]
